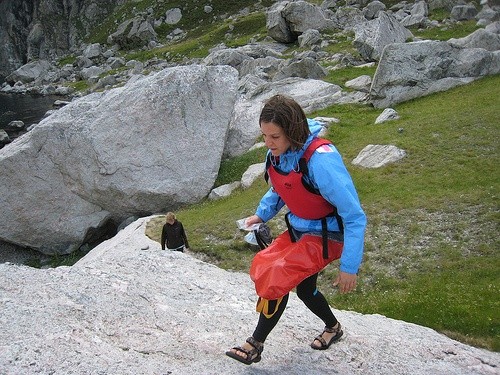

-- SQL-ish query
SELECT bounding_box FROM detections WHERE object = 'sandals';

[226,337,264,365]
[311,322,343,350]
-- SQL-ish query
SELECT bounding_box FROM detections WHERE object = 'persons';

[224,94,369,366]
[160,212,191,254]
